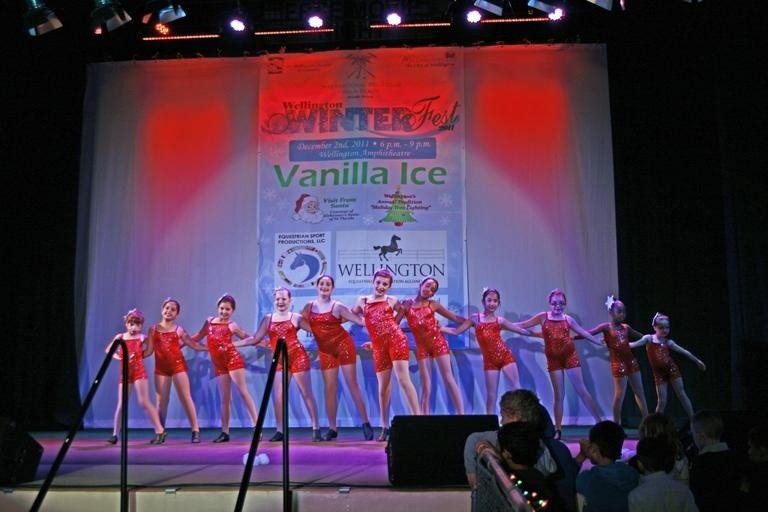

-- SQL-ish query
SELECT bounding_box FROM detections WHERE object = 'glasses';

[24,6,61,37]
[90,1,134,36]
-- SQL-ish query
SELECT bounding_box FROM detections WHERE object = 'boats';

[385,415,499,490]
[0,422,45,488]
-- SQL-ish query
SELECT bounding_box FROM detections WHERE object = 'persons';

[440,287,543,414]
[571,294,649,425]
[501,289,607,440]
[574,420,640,512]
[463,389,557,487]
[600,313,707,416]
[739,424,767,512]
[627,435,699,512]
[689,408,742,512]
[264,275,375,442]
[627,412,683,475]
[361,277,467,415]
[232,287,322,443]
[142,297,208,443]
[341,269,421,441]
[470,420,579,512]
[179,292,271,444]
[105,307,167,445]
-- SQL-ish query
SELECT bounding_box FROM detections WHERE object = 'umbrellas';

[312,426,321,442]
[192,431,200,443]
[213,432,229,443]
[362,422,373,440]
[323,429,337,441]
[554,428,561,440]
[269,432,283,441]
[252,426,263,441]
[106,436,117,444]
[376,427,390,442]
[150,438,165,443]
[151,431,167,444]
